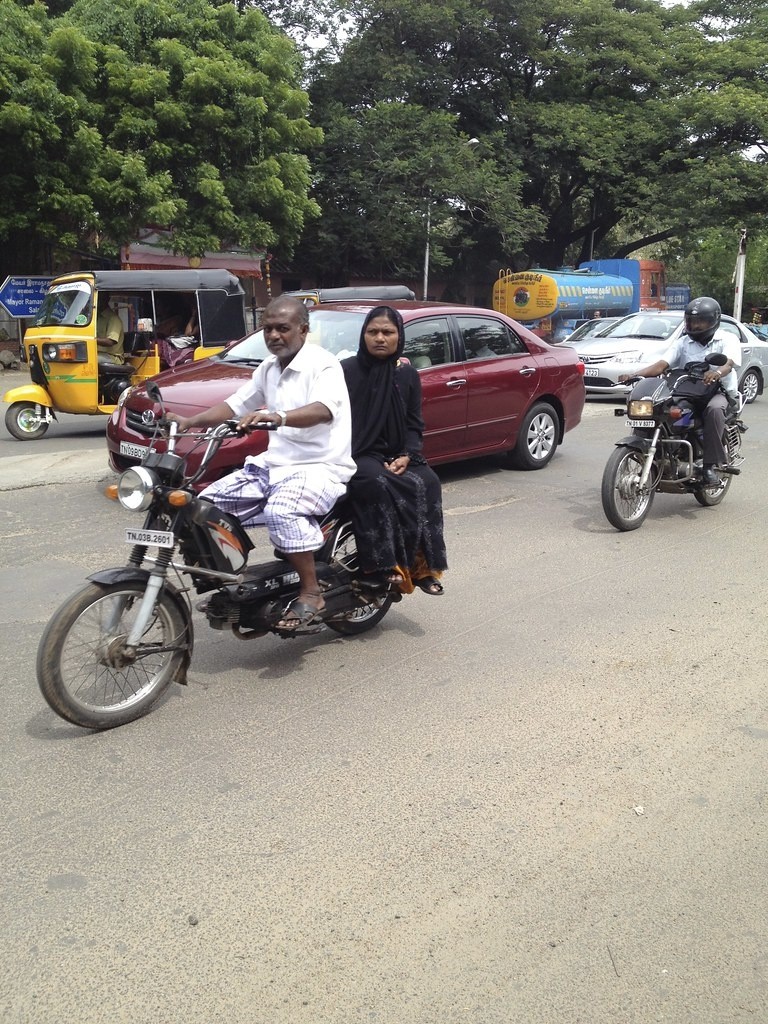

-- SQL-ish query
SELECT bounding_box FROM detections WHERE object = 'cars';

[553,316,627,369]
[105,298,587,515]
[570,309,768,403]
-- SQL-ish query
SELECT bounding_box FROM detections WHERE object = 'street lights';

[423,137,483,306]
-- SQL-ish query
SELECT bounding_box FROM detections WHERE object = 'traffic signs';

[0,272,71,321]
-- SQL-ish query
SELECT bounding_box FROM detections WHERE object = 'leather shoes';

[704,467,719,485]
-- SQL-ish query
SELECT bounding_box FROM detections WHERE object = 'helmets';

[684,297,722,340]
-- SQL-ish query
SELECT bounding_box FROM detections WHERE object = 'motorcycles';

[5,267,247,447]
[34,377,420,730]
[601,352,749,534]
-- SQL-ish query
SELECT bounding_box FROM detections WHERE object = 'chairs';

[637,321,668,340]
[402,335,433,371]
[99,332,140,383]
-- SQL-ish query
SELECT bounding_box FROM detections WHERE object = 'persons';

[463,326,497,360]
[619,298,744,486]
[339,305,448,598]
[159,296,209,368]
[593,310,602,319]
[96,291,126,365]
[159,296,359,631]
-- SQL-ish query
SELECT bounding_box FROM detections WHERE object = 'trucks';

[494,258,669,346]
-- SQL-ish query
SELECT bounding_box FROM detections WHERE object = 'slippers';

[384,575,444,595]
[275,602,327,631]
